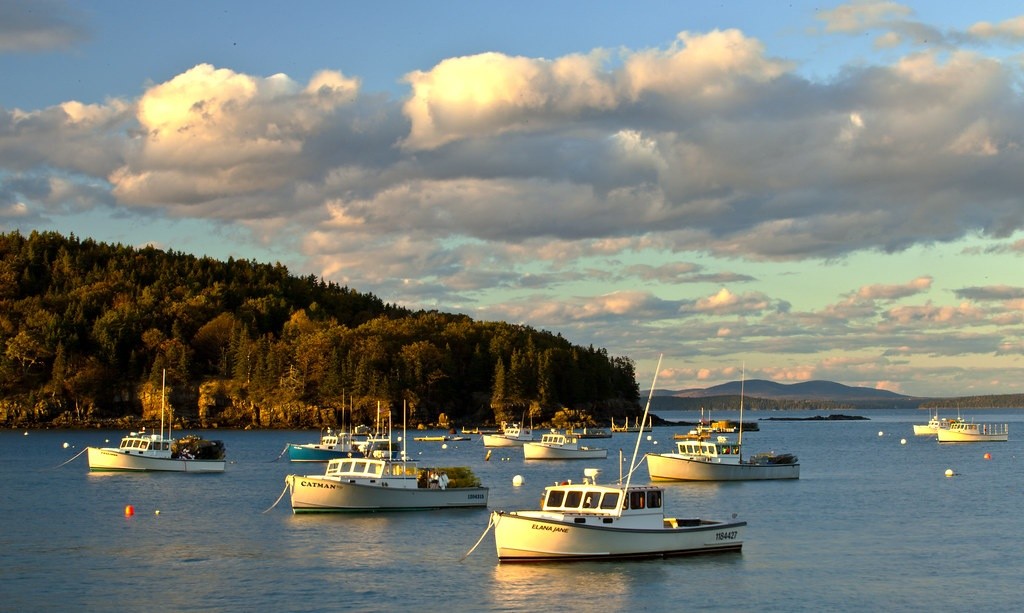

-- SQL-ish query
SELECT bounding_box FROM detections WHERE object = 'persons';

[430,471,439,489]
[439,471,448,490]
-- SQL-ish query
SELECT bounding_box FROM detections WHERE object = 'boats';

[551,424,614,439]
[937,403,1009,443]
[647,362,801,483]
[283,398,490,514]
[523,425,609,461]
[913,402,965,437]
[482,411,542,448]
[609,414,653,433]
[670,406,739,442]
[287,387,403,464]
[87,367,227,474]
[491,352,750,564]
